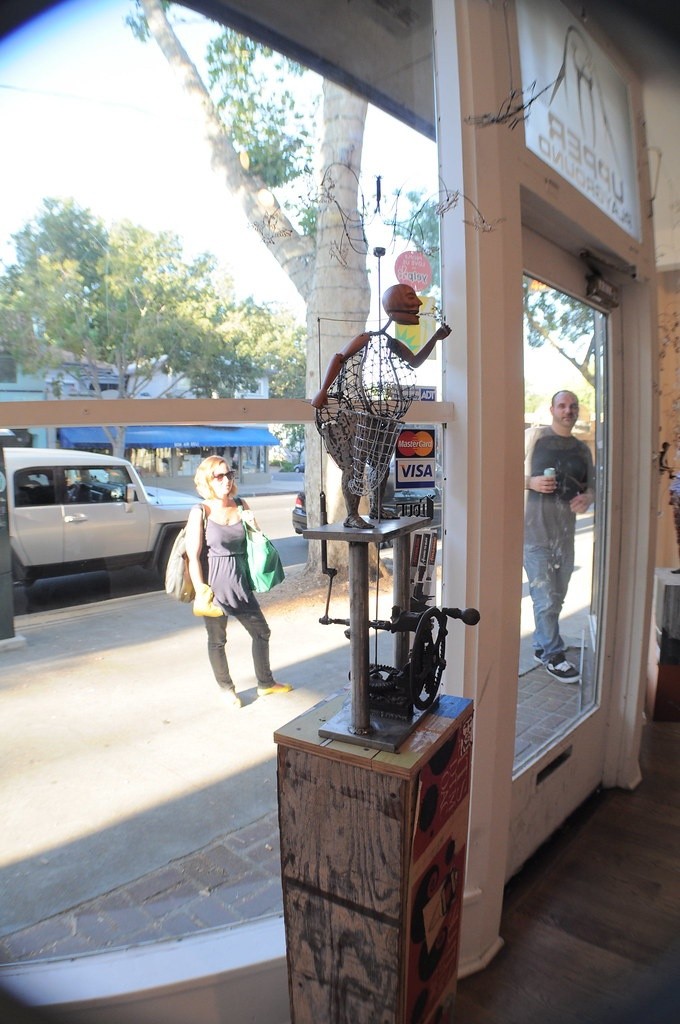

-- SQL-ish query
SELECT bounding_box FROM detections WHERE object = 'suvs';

[2,447,206,589]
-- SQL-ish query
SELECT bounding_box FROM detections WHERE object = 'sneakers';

[533,648,581,683]
[257,682,293,695]
[223,691,242,710]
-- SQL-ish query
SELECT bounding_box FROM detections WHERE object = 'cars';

[292,461,441,548]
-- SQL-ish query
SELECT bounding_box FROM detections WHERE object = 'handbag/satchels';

[164,529,196,604]
[244,527,286,593]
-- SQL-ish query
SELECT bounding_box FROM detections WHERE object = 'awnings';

[58,424,283,449]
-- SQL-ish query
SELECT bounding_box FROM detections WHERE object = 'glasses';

[213,470,236,482]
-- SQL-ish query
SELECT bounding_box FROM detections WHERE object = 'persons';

[183,456,292,707]
[522,389,597,684]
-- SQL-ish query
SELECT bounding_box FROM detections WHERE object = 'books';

[406,528,440,600]
